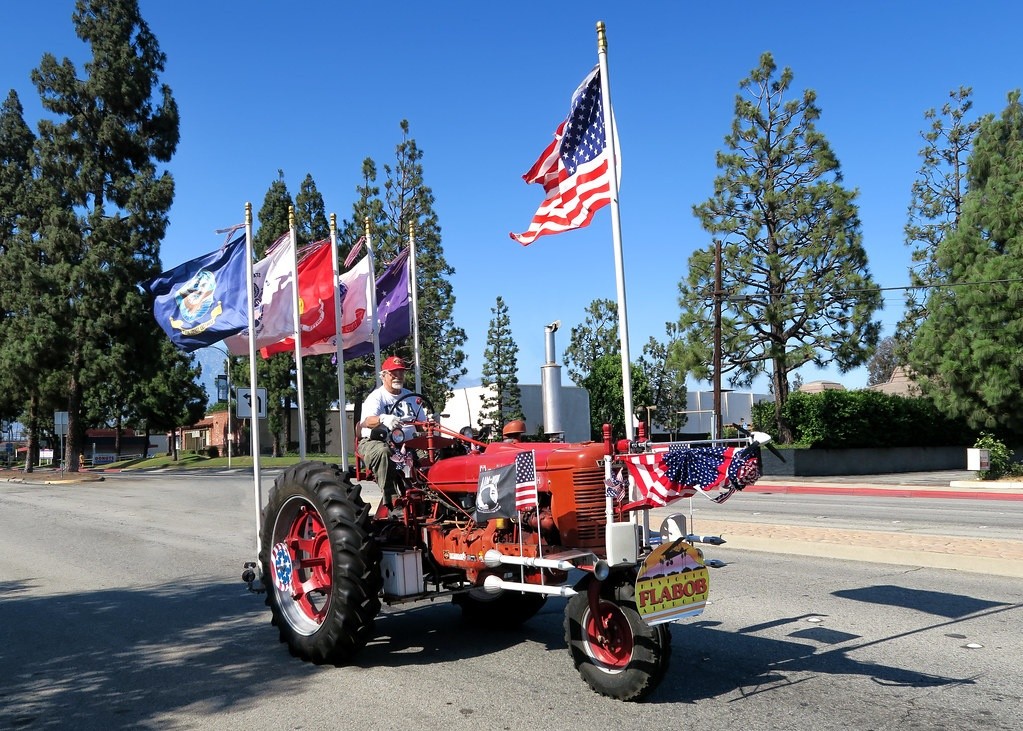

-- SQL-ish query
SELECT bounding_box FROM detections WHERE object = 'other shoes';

[387,506,405,519]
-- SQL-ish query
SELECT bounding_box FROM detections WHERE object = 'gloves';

[428,412,441,424]
[378,413,405,431]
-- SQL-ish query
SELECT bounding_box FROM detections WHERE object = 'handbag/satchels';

[370,429,387,440]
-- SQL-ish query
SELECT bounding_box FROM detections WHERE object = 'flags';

[139,235,248,353]
[510,67,620,246]
[261,238,337,360]
[516,451,538,510]
[222,237,295,356]
[293,257,373,361]
[332,247,413,362]
[476,465,519,523]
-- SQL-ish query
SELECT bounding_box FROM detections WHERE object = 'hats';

[382,356,406,370]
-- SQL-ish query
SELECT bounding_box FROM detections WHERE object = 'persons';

[79,452,84,469]
[739,418,745,428]
[357,357,429,516]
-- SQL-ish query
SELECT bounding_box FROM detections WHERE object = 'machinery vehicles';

[242,321,726,701]
